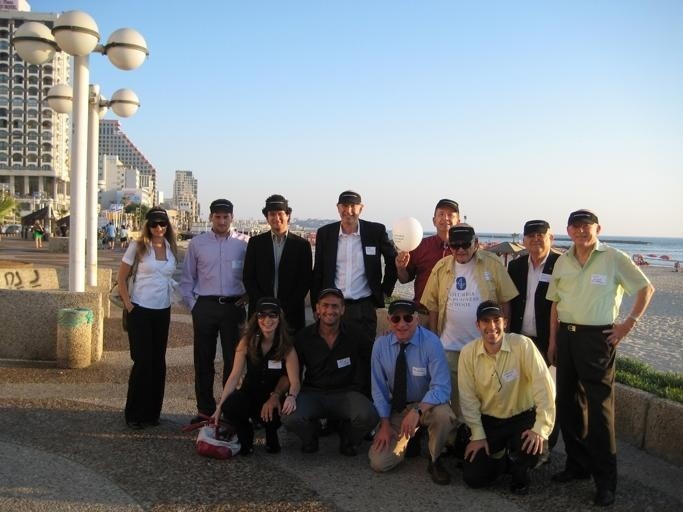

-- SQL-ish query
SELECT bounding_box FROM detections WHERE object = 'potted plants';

[614,356,657,451]
[644,372,682,466]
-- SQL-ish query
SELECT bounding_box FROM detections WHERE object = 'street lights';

[461,214,466,223]
[8,12,148,297]
[43,81,143,290]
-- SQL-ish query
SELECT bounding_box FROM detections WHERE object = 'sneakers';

[191,416,204,423]
[266,431,280,454]
[128,421,145,429]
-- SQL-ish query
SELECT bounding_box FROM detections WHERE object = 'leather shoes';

[428,462,449,484]
[336,422,357,456]
[595,488,616,506]
[551,468,590,483]
[512,474,529,495]
[303,435,318,453]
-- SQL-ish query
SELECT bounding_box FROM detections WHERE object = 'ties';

[393,342,411,414]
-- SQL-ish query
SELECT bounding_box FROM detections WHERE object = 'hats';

[480,307,503,321]
[436,200,458,211]
[338,192,361,204]
[265,199,287,210]
[319,288,343,299]
[388,300,419,315]
[568,212,598,226]
[210,202,233,213]
[524,220,550,235]
[148,210,166,220]
[449,228,475,241]
[256,299,278,312]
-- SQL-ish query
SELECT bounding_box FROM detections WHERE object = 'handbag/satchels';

[109,272,135,309]
[196,421,241,458]
[466,440,530,482]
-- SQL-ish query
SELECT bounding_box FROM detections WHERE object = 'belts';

[560,321,576,331]
[214,296,241,304]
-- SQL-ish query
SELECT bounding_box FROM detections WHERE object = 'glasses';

[149,220,166,228]
[391,315,413,323]
[450,242,471,251]
[256,312,277,318]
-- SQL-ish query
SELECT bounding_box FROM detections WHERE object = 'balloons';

[392,216,423,251]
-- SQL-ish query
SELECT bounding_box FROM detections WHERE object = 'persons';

[420,222,521,456]
[395,198,461,329]
[260,287,381,456]
[457,299,558,494]
[313,189,398,442]
[507,218,563,370]
[32,219,43,249]
[369,296,456,484]
[116,207,180,430]
[180,199,253,425]
[102,221,128,250]
[544,208,656,508]
[210,297,303,454]
[242,193,314,383]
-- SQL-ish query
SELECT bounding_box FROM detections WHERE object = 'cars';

[177,231,201,240]
[1,224,21,233]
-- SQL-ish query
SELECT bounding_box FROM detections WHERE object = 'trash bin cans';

[56,307,93,369]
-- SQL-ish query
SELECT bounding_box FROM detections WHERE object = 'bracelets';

[412,403,423,417]
[285,391,297,399]
[269,390,281,395]
[628,313,639,322]
